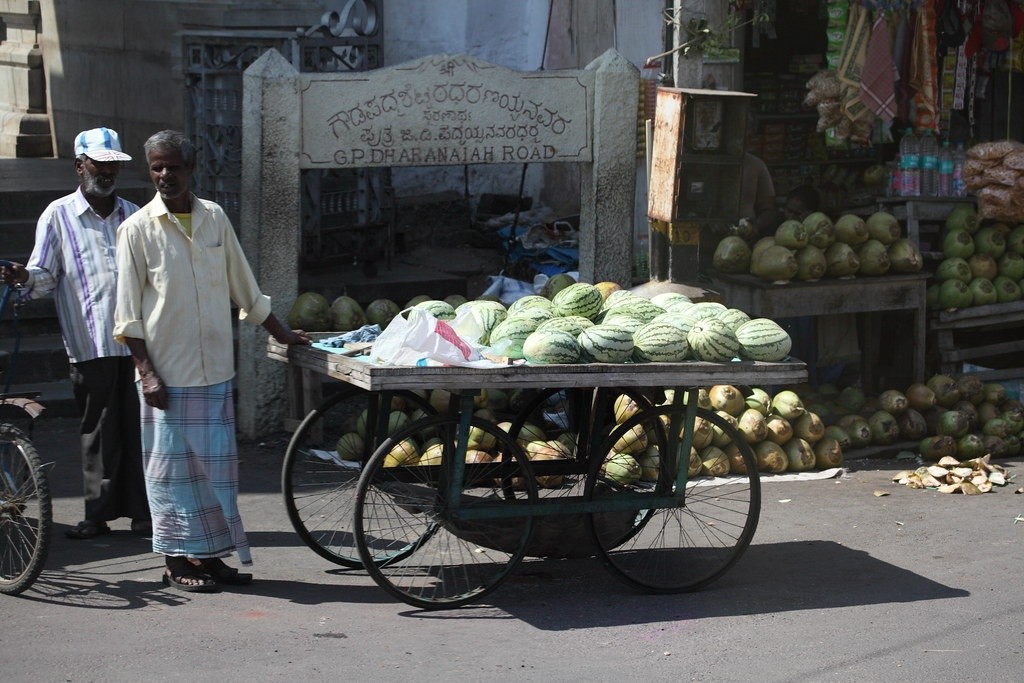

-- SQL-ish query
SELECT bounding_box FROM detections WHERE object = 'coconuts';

[287,291,504,333]
[336,385,843,479]
[927,205,1024,309]
[795,373,1024,457]
[714,211,922,279]
[542,274,578,299]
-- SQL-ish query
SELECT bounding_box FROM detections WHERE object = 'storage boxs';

[744,55,826,190]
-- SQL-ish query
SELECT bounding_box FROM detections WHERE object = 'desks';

[705,272,933,399]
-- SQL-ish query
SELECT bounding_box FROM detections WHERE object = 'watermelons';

[450,284,791,365]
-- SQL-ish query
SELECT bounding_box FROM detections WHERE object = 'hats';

[74,127,133,162]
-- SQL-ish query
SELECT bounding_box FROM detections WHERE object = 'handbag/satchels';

[981,0,1011,50]
[937,5,965,47]
[368,306,526,370]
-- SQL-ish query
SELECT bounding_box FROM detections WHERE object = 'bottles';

[900,127,921,196]
[920,129,940,197]
[952,142,967,199]
[938,141,955,198]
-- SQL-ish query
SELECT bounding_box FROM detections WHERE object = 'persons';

[714,112,820,397]
[113,130,311,591]
[0,127,153,538]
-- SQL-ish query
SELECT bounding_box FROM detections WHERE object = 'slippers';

[196,558,252,585]
[63,522,110,539]
[131,521,152,535]
[162,568,218,592]
